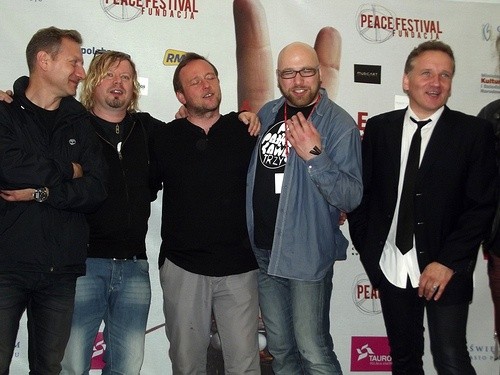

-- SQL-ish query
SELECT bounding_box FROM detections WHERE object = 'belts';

[112,254,147,261]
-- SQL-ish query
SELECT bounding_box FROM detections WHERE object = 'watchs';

[34,187,47,202]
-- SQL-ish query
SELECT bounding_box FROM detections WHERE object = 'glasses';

[278,69,319,79]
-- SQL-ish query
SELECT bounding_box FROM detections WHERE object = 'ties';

[395,117,432,255]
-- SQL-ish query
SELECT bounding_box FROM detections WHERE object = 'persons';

[0,50,261,375]
[345,40,500,375]
[0,26,107,375]
[175,42,363,375]
[476,98,500,345]
[158,52,347,375]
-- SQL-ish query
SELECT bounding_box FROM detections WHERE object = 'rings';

[433,285,438,290]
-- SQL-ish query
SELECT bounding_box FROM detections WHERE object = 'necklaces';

[92,109,129,134]
[284,94,321,157]
